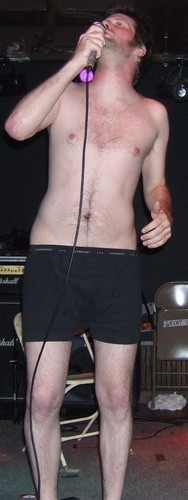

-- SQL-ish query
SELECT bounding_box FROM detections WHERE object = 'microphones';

[86,20,105,74]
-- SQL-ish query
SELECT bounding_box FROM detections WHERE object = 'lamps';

[158,76,188,102]
[0,64,26,96]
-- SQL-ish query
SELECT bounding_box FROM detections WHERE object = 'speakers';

[0,264,97,417]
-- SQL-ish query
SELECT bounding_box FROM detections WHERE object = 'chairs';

[151,281,188,409]
[14,313,100,472]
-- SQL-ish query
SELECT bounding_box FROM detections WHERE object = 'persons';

[6,5,173,500]
